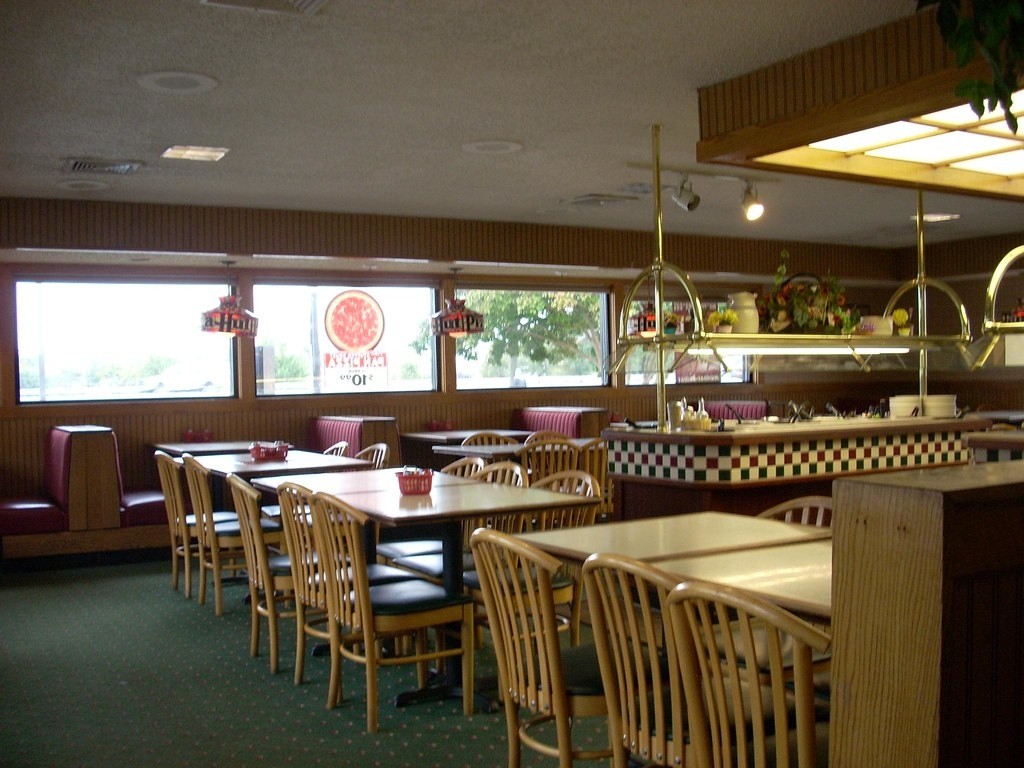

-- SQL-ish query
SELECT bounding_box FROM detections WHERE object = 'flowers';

[891,307,909,326]
[768,249,862,335]
[707,309,739,326]
[664,310,684,331]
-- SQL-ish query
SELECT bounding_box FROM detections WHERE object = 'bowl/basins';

[641,332,656,338]
[859,316,893,339]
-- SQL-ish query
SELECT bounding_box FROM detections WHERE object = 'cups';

[700,418,712,431]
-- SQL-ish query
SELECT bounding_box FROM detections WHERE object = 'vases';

[717,323,733,333]
[665,326,676,334]
[898,327,910,338]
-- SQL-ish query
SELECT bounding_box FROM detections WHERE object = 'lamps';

[672,171,700,212]
[632,275,656,339]
[429,267,484,340]
[738,180,765,221]
[201,260,259,339]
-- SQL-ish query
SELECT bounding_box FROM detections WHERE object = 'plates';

[924,396,956,417]
[889,395,922,417]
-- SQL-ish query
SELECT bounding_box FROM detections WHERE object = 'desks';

[647,538,831,620]
[513,511,831,695]
[828,461,1024,768]
[194,450,374,603]
[148,440,296,588]
[251,467,482,656]
[400,431,531,445]
[430,440,609,459]
[334,482,602,713]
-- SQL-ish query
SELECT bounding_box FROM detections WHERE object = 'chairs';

[469,496,834,768]
[154,430,613,734]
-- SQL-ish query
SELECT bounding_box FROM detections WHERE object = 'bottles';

[646,303,656,331]
[696,398,709,430]
[907,308,917,336]
[681,396,691,428]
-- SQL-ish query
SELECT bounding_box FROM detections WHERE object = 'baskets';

[249,445,288,460]
[182,431,212,442]
[427,421,455,431]
[396,469,433,494]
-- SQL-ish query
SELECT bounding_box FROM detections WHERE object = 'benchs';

[0,425,183,574]
[511,407,614,439]
[304,416,403,467]
[704,400,783,420]
